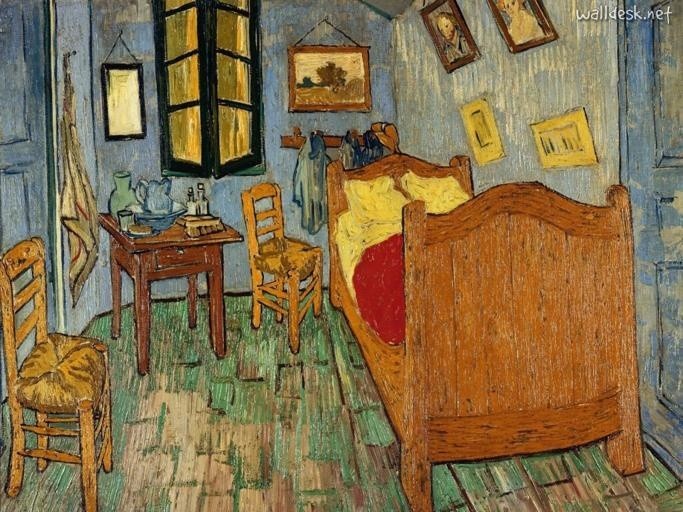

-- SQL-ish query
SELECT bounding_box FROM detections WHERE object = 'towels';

[58,53,101,309]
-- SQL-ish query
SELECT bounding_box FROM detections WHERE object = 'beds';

[327,155,646,511]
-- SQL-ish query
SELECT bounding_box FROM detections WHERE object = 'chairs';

[242,184,322,354]
[3,237,114,510]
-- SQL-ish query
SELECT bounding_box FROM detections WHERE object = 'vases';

[111,170,135,216]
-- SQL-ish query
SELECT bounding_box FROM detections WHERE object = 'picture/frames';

[459,99,506,164]
[532,107,599,171]
[489,1,559,55]
[287,46,372,112]
[420,0,481,75]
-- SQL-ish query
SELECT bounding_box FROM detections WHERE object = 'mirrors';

[102,63,147,141]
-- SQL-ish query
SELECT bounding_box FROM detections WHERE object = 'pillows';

[343,175,404,227]
[401,171,470,217]
